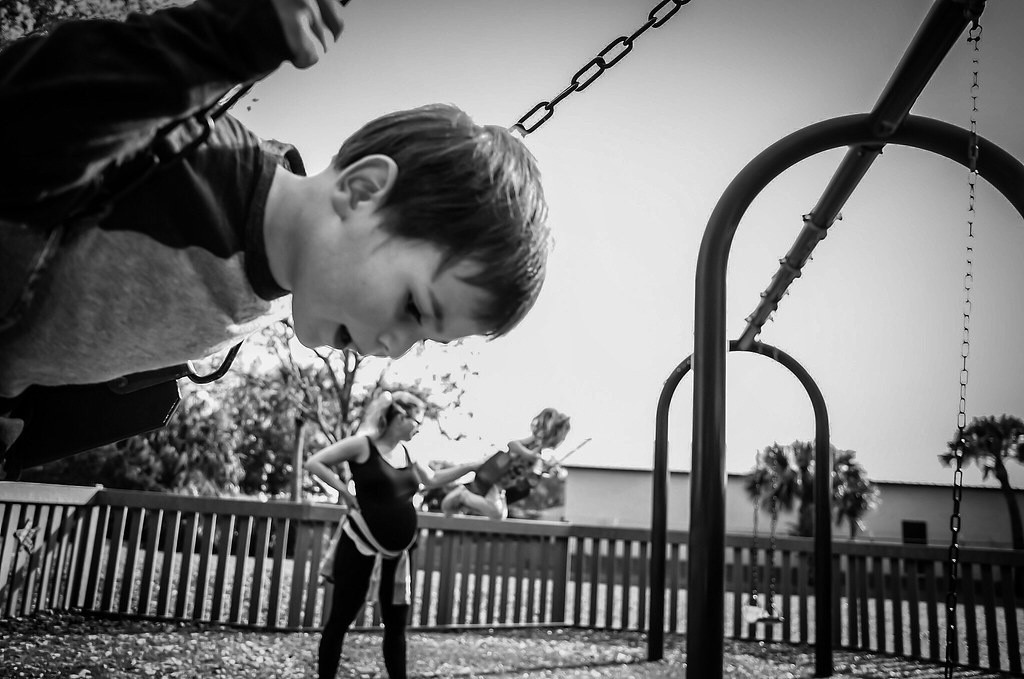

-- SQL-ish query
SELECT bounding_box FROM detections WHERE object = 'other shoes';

[441,484,468,513]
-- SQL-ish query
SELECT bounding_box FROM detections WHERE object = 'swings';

[2,1,695,480]
[740,313,787,626]
[477,348,740,507]
[941,24,983,679]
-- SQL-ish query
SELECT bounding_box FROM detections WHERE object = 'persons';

[428,407,570,521]
[0,0,549,400]
[304,391,482,679]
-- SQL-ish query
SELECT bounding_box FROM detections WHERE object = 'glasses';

[404,409,424,428]
